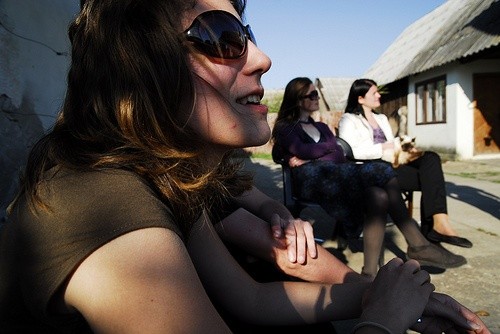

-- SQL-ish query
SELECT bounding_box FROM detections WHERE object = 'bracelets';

[351,321,393,333]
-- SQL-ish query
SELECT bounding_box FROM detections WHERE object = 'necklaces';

[300,120,312,124]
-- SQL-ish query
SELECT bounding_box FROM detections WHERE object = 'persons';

[337,77,474,250]
[0,0,493,334]
[272,77,467,281]
[203,175,361,285]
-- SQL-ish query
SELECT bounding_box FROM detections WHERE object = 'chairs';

[282,136,354,246]
[384,143,425,229]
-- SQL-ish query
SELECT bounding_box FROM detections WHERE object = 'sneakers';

[406,243,466,268]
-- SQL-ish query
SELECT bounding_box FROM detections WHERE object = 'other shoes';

[428,230,472,248]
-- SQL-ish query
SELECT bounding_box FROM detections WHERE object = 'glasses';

[304,90,318,100]
[176,10,257,59]
